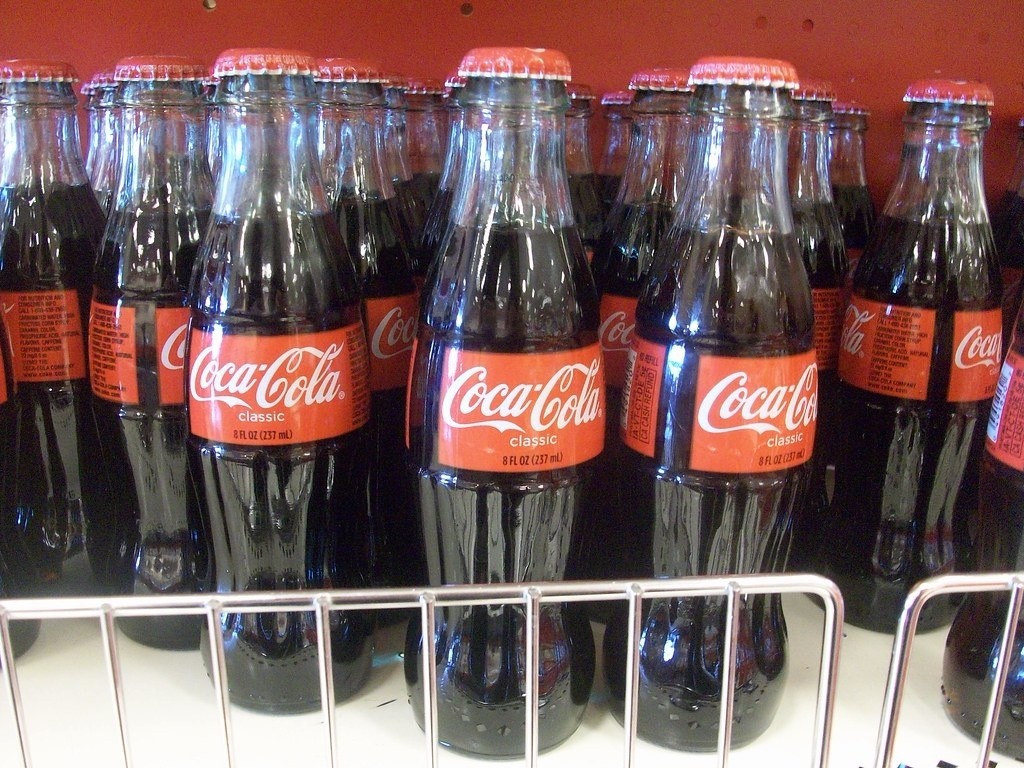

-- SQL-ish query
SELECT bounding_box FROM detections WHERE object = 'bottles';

[600,56,822,755]
[0,58,1001,653]
[190,47,377,715]
[937,290,1024,759]
[404,43,604,756]
[991,118,1024,330]
